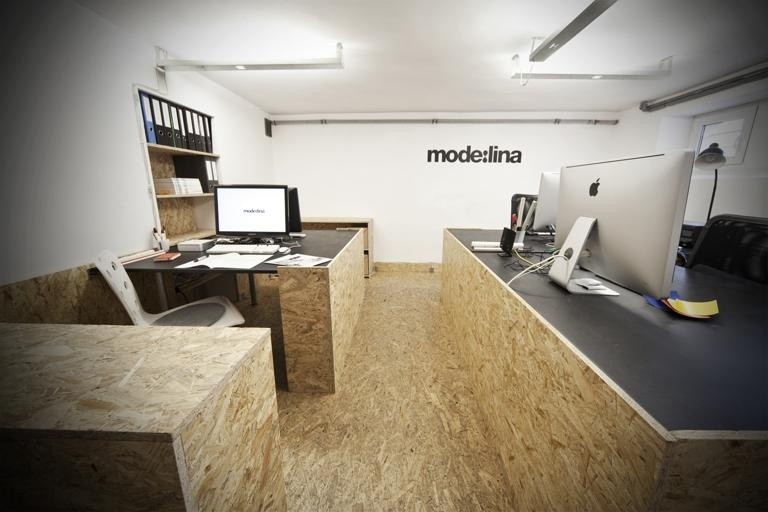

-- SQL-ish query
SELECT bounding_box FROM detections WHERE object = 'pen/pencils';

[194,253,209,262]
[153,225,166,249]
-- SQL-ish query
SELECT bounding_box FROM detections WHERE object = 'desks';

[0,322,289,512]
[86,229,366,395]
[441,225,768,512]
[300,216,374,278]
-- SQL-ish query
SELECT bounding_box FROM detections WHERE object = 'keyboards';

[289,233,306,239]
[206,244,280,254]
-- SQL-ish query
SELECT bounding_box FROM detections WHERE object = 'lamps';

[513,54,674,79]
[639,66,768,113]
[694,142,727,224]
[529,0,618,63]
[153,41,344,71]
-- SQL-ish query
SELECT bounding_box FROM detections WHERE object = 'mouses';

[279,247,288,253]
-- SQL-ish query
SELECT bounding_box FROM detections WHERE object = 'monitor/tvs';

[287,187,301,232]
[214,185,287,244]
[532,172,561,247]
[548,150,695,300]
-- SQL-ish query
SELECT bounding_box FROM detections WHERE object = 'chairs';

[511,194,538,231]
[684,212,768,289]
[94,247,246,327]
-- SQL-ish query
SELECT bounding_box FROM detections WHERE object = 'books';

[151,252,182,261]
[154,175,204,196]
[173,252,273,269]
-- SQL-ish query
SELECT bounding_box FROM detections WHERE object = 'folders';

[141,92,212,153]
[173,155,218,193]
